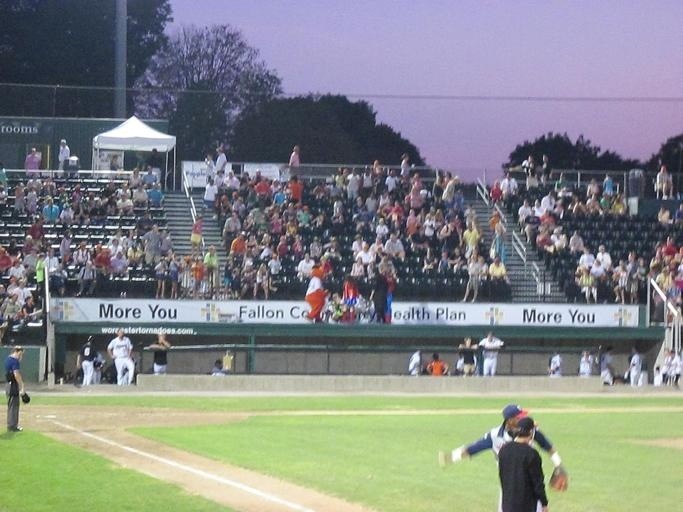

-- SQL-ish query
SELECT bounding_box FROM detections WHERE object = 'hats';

[514,417,540,429]
[501,404,528,419]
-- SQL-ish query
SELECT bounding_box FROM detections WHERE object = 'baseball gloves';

[550,467,567,491]
[22,393,30,404]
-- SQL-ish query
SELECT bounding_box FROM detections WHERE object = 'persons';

[209,348,235,375]
[179,146,683,327]
[438,401,568,512]
[1,139,179,341]
[547,345,683,386]
[498,416,549,512]
[71,329,171,386]
[408,330,504,376]
[4,345,26,432]
